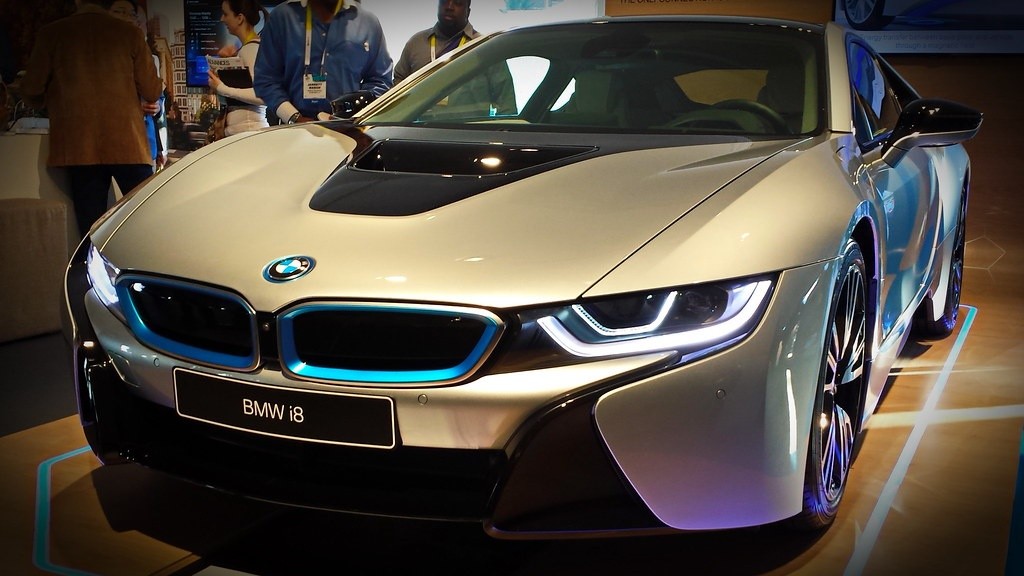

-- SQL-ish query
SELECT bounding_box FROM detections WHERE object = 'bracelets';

[289,113,299,124]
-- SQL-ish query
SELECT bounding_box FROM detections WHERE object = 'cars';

[68,18,984,552]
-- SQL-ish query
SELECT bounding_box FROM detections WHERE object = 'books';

[205,54,255,107]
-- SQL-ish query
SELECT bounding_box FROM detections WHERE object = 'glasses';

[113,8,138,19]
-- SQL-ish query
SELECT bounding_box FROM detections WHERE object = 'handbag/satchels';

[203,108,227,145]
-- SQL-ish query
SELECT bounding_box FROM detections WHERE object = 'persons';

[252,0,390,126]
[393,0,520,117]
[22,0,168,238]
[206,0,270,139]
